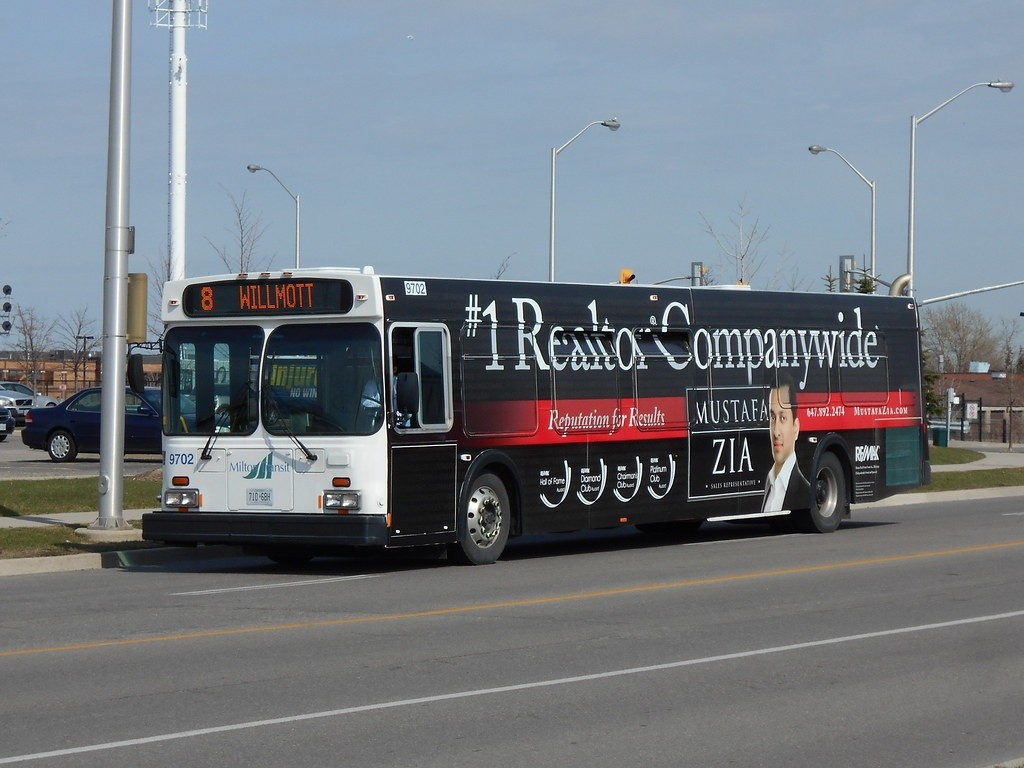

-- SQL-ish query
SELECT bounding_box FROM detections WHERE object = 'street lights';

[809,142,876,294]
[245,164,301,269]
[906,79,1014,298]
[550,118,621,285]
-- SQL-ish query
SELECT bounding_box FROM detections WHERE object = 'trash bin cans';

[931,428,947,447]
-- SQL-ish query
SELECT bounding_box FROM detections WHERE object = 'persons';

[761,370,811,512]
[361,352,411,427]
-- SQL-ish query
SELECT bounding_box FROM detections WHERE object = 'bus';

[140,263,935,564]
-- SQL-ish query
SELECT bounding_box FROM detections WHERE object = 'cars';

[0,380,59,442]
[21,385,230,463]
